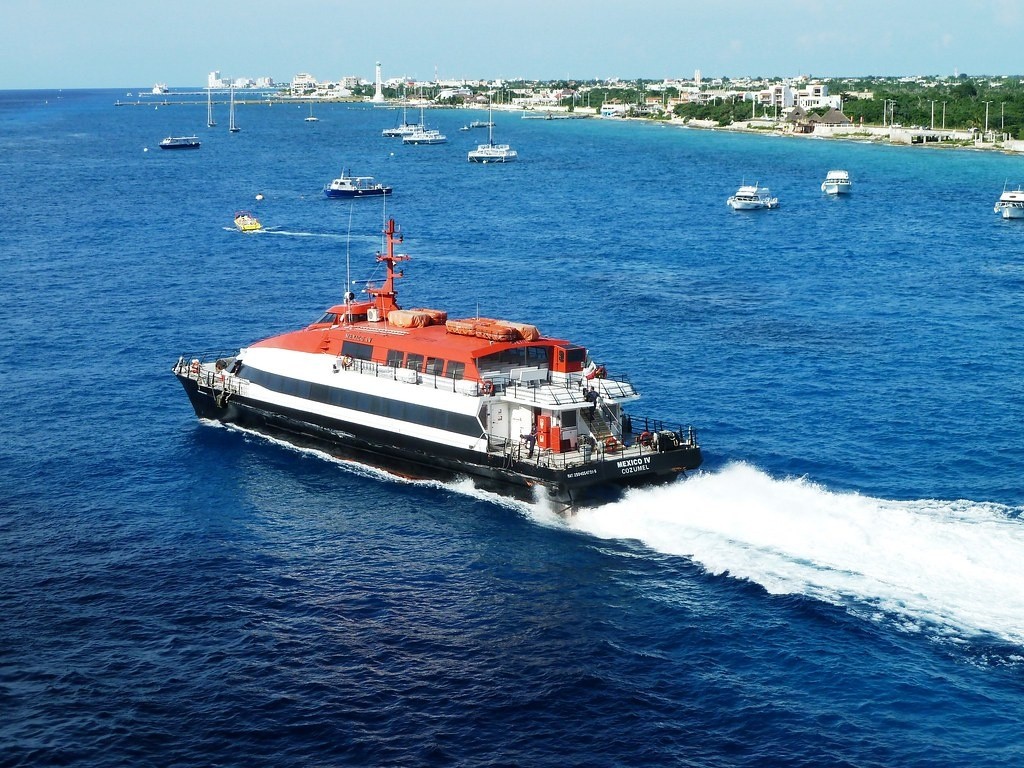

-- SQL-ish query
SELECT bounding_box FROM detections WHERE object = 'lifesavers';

[598,368,606,378]
[640,432,651,445]
[481,380,494,394]
[216,394,229,409]
[345,354,353,367]
[606,437,617,451]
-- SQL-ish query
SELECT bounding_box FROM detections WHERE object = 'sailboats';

[466,85,518,164]
[205,85,219,127]
[304,95,320,121]
[381,73,422,137]
[227,75,242,133]
[402,82,448,145]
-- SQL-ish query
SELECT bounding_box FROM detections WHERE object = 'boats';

[157,134,201,149]
[726,175,780,211]
[169,192,706,516]
[821,170,852,195]
[233,209,262,232]
[387,306,540,341]
[994,177,1024,220]
[322,166,394,198]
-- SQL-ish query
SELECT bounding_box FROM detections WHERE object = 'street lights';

[941,101,947,129]
[927,99,938,130]
[880,98,891,129]
[889,100,898,125]
[981,100,995,132]
[1000,102,1007,129]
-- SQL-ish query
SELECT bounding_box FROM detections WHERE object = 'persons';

[586,387,601,419]
[520,434,536,458]
[530,423,538,439]
